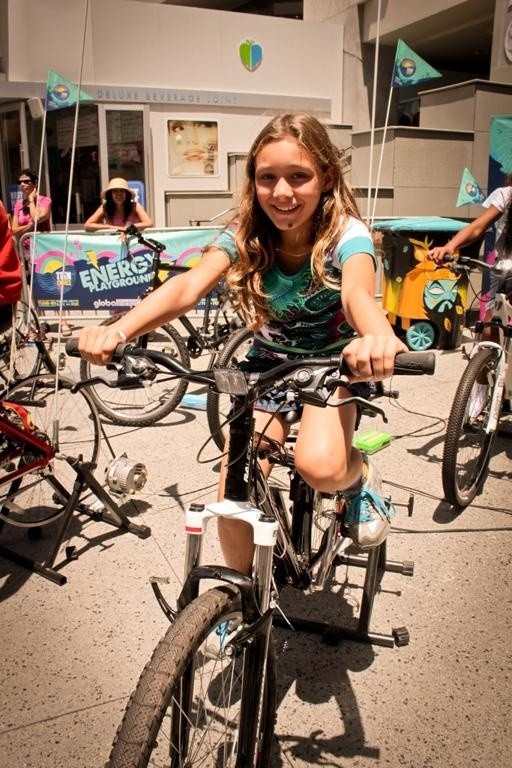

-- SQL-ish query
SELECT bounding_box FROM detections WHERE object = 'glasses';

[17,180,33,185]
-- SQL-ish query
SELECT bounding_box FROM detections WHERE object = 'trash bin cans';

[373,217,472,350]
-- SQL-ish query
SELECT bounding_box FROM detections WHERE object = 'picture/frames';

[160,103,234,195]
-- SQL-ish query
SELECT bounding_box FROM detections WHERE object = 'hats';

[100,177,136,200]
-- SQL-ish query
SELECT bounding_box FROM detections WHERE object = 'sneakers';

[342,452,394,550]
[203,619,238,660]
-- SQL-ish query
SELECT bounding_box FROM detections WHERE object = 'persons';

[424,170,511,433]
[1,198,24,336]
[13,167,54,270]
[83,177,154,235]
[74,109,414,662]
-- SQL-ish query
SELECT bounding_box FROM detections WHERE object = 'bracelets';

[29,200,35,203]
[118,328,127,344]
